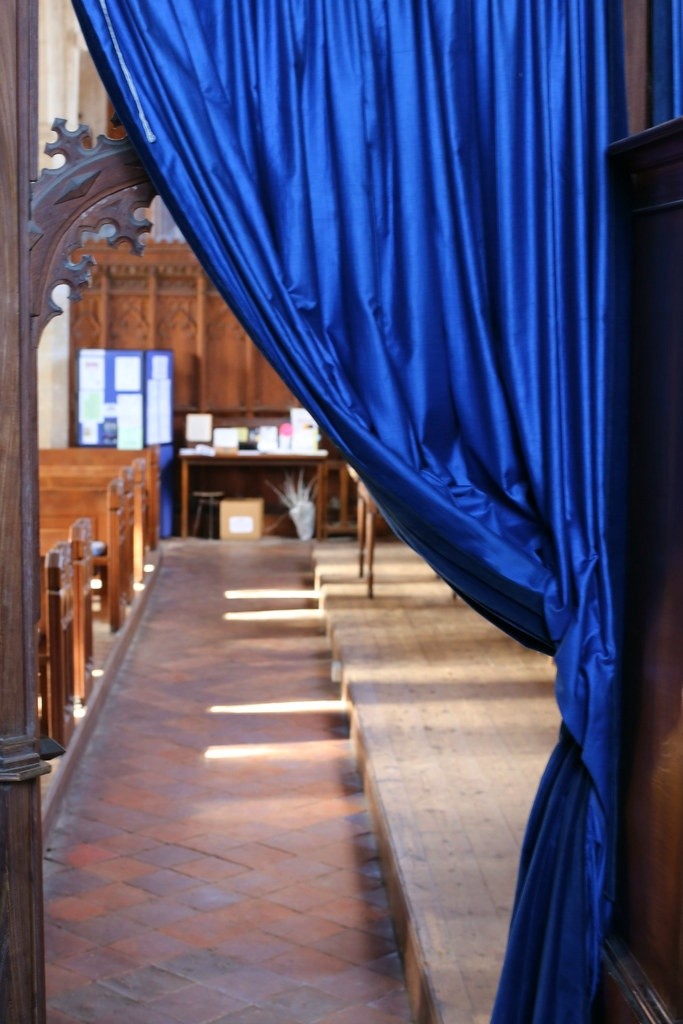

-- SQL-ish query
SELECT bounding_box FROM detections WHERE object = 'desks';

[345,464,461,601]
[180,447,329,542]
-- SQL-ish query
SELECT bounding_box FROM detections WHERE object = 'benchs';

[37,446,160,758]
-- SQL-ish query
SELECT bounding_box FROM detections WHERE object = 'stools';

[192,491,224,539]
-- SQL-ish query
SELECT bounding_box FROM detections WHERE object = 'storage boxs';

[219,496,264,540]
